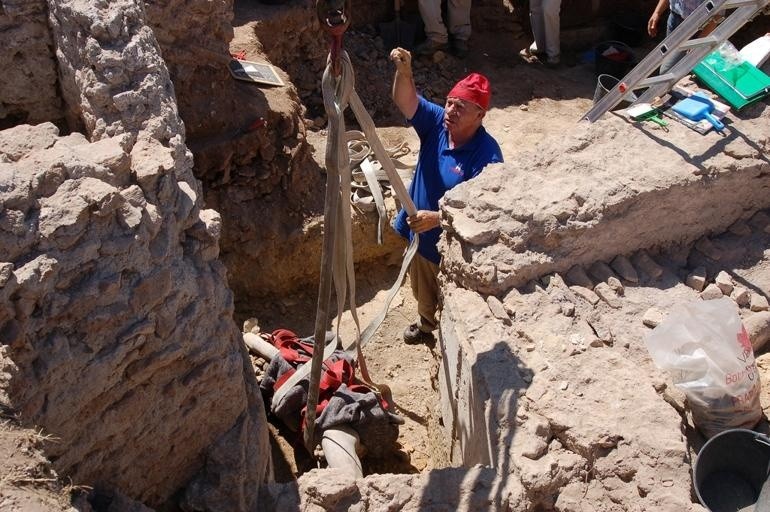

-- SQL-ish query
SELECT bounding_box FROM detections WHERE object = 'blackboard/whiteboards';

[226,59,286,86]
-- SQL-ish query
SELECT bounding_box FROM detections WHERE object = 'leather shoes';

[403,323,431,345]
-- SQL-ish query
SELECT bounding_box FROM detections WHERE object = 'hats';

[447,72,490,111]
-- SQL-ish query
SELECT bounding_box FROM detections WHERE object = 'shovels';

[381,0,416,52]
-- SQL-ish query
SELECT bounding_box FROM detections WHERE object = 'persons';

[390,47,503,342]
[647,1,726,76]
[529,1,561,70]
[418,1,471,59]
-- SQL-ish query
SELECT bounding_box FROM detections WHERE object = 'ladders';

[579,0,770,122]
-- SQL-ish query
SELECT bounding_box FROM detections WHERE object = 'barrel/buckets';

[594,74,637,111]
[596,40,635,76]
[694,428,770,512]
